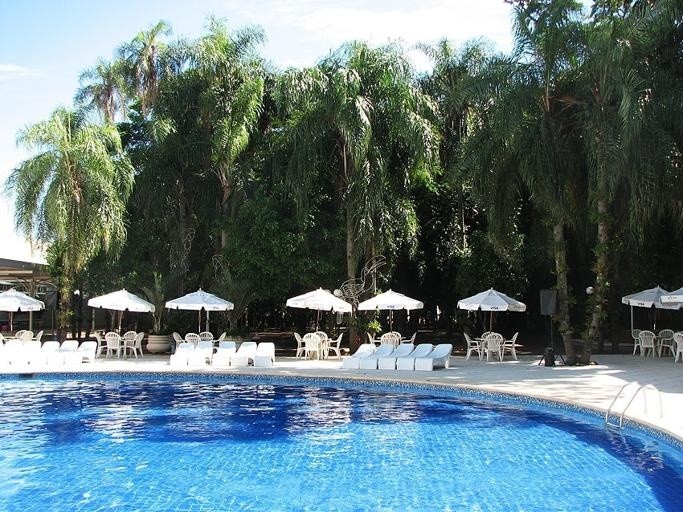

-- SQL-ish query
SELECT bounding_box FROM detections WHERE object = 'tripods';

[538,315,566,366]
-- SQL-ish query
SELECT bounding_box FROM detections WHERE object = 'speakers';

[45,291,60,311]
[540,289,559,315]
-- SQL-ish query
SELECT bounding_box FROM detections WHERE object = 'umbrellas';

[357,289,424,332]
[88,288,155,334]
[165,288,235,335]
[658,287,683,302]
[286,287,353,332]
[0,288,46,336]
[622,287,681,329]
[456,287,526,334]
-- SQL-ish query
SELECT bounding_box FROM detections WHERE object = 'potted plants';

[140,270,172,354]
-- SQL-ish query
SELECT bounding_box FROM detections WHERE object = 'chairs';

[341,330,454,372]
[168,330,277,369]
[293,330,344,363]
[621,284,681,363]
[462,330,522,364]
[1,330,145,367]
